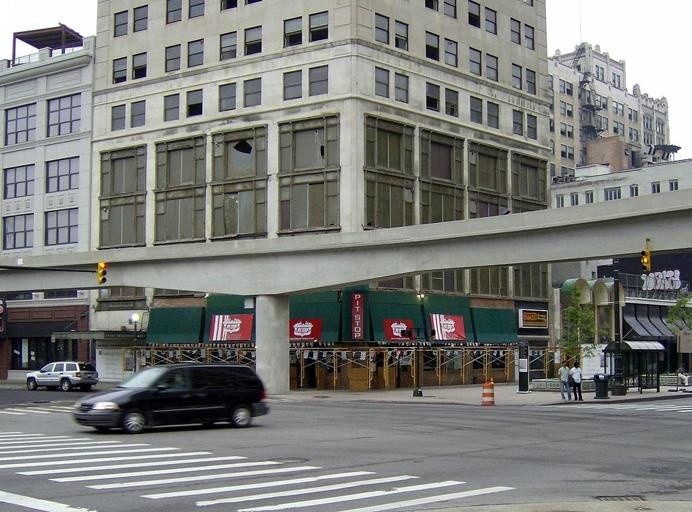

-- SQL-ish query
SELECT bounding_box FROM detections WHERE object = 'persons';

[558,360,571,401]
[569,361,584,401]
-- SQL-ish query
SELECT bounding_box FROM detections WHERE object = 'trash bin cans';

[593,373,610,399]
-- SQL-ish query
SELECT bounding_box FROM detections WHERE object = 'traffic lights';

[640,249,653,272]
[97,261,107,285]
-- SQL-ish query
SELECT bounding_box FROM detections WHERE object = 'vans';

[70,363,274,435]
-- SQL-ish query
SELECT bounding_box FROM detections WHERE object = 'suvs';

[25,360,99,393]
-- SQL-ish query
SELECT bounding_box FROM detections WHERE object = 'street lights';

[130,311,142,371]
